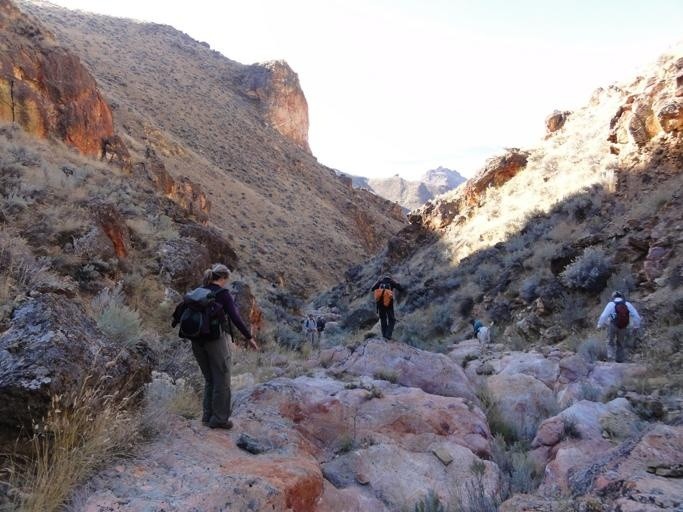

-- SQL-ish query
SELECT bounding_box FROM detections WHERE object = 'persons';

[469,318,484,337]
[305,314,316,343]
[315,315,326,342]
[596,290,640,363]
[370,272,403,339]
[172,264,258,429]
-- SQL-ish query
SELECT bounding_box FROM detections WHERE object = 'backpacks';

[178,288,228,339]
[610,299,629,329]
[373,283,392,306]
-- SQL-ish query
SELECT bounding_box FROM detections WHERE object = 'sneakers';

[201,419,234,429]
